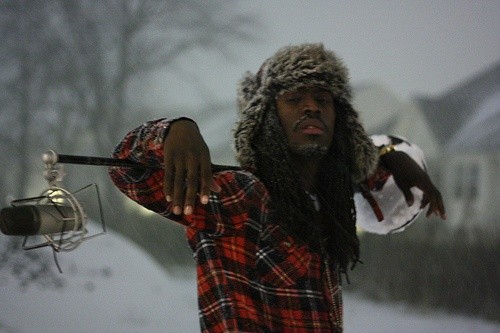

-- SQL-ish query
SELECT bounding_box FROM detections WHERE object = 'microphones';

[0,205,87,236]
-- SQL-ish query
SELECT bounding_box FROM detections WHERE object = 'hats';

[229,41,379,186]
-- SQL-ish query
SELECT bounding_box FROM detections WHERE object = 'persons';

[108,42,446,333]
